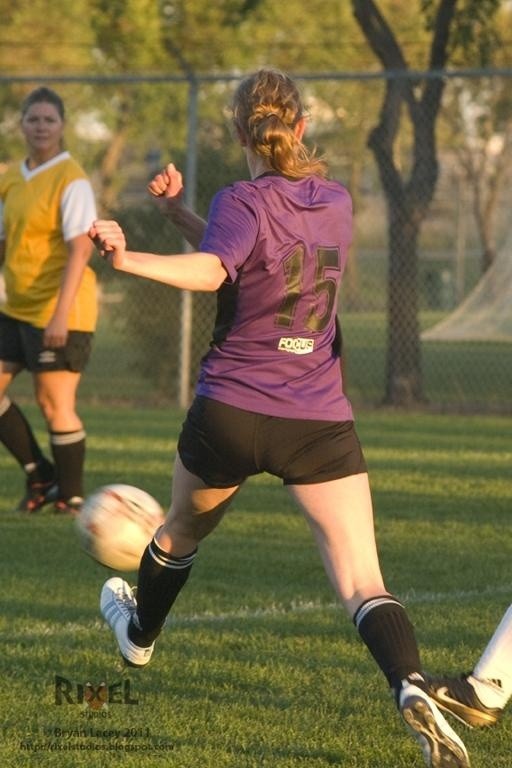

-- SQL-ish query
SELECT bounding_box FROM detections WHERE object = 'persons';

[80,62,476,768]
[418,598,512,735]
[2,83,105,516]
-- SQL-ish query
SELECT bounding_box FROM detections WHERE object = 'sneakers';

[14,471,83,517]
[99,577,157,669]
[397,673,504,767]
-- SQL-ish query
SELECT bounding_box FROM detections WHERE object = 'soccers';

[72,484,166,571]
